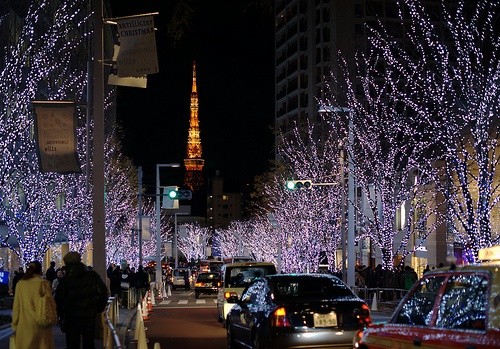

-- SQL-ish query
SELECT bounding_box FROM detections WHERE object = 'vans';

[172,269,192,291]
[215,263,278,329]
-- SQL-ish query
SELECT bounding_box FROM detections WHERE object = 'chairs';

[231,275,242,287]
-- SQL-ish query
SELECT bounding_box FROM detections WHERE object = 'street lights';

[156,164,183,282]
[318,105,355,287]
[174,213,189,268]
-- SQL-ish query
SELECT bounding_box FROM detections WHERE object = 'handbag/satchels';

[35,280,57,327]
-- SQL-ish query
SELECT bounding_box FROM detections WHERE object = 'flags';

[160,185,180,210]
[31,98,82,174]
[116,16,159,78]
[107,33,148,89]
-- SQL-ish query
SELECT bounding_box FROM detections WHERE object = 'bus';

[200,260,225,273]
[149,263,170,281]
[224,257,255,280]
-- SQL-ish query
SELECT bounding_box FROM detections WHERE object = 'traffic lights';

[170,191,192,200]
[286,180,312,190]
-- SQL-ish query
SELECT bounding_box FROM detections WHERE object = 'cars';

[354,245,500,349]
[225,274,371,349]
[193,273,222,301]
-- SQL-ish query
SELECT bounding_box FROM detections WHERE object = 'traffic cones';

[141,299,151,322]
[156,287,163,300]
[154,343,160,349]
[371,293,379,311]
[131,303,149,343]
[147,293,152,312]
[149,286,156,304]
[162,283,167,297]
[136,321,148,349]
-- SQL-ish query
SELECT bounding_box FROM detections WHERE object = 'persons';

[53,250,109,349]
[107,255,225,311]
[250,271,262,281]
[230,272,244,286]
[11,260,59,349]
[280,254,467,309]
[0,257,95,313]
